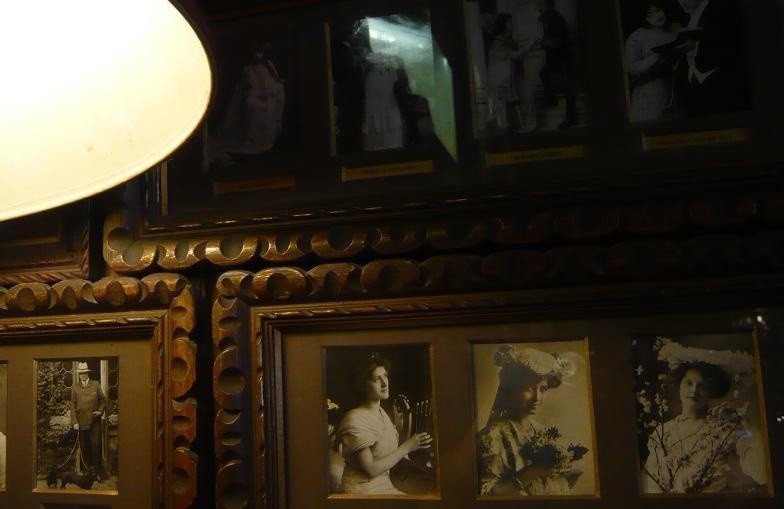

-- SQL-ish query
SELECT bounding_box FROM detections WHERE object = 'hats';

[657,341,755,375]
[493,345,565,389]
[75,361,91,373]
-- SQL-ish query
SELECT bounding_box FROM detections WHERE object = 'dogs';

[45,466,57,488]
[56,473,94,489]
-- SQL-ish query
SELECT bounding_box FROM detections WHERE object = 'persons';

[531,1,582,134]
[635,339,768,494]
[67,361,110,483]
[650,0,748,121]
[487,9,537,136]
[475,343,587,495]
[621,3,670,137]
[235,39,288,153]
[336,352,435,498]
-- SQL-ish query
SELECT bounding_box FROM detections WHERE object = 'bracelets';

[514,474,526,491]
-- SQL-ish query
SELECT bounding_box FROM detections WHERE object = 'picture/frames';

[0,194,98,277]
[209,240,784,507]
[104,3,783,275]
[1,271,195,508]
